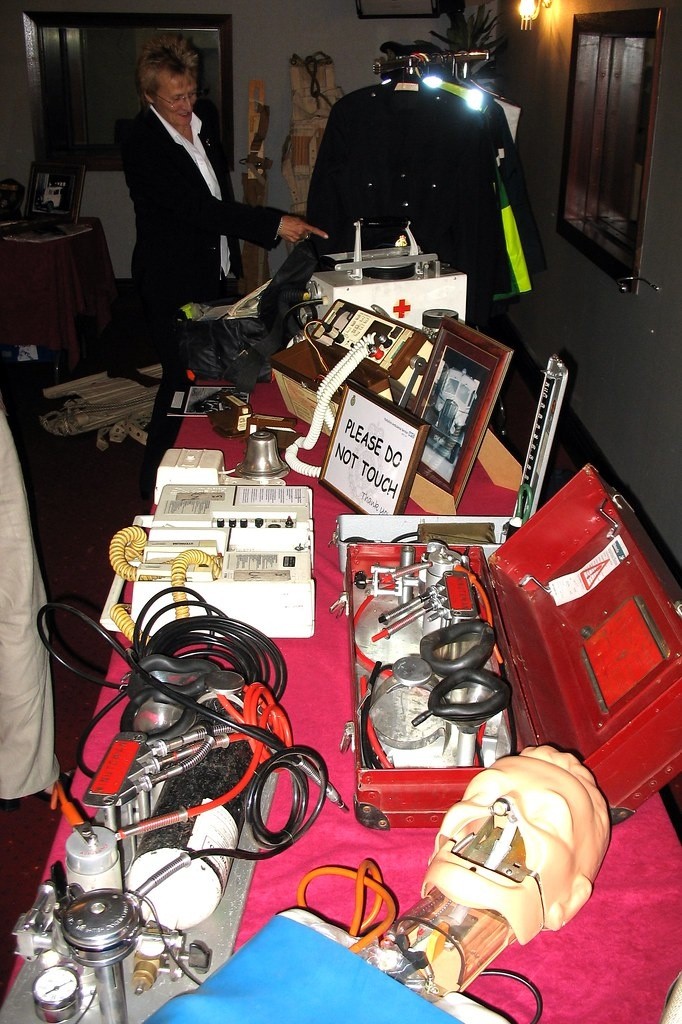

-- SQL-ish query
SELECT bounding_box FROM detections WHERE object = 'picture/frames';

[24,161,86,225]
[411,315,515,512]
[22,11,234,171]
[317,377,431,515]
[356,0,440,19]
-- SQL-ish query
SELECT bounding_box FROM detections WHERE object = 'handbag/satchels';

[188,316,281,393]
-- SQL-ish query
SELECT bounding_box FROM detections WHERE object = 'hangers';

[395,50,523,113]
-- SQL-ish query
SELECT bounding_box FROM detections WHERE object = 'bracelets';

[275,216,283,242]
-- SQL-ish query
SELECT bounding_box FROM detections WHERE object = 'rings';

[304,234,310,241]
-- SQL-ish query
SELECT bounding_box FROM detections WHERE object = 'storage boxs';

[337,354,568,573]
[346,462,682,829]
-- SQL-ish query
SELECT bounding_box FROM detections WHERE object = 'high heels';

[0,770,72,812]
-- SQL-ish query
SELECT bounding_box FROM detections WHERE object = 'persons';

[113,36,333,500]
[0,398,62,800]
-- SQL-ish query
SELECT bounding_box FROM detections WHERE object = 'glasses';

[155,89,201,108]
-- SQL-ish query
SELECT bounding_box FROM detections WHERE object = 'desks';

[0,218,118,385]
